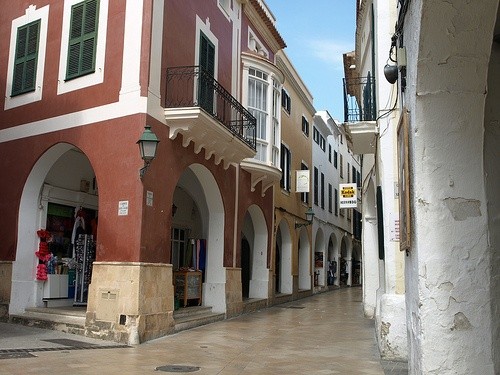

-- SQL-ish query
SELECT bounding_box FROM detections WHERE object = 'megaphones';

[384,64,398,84]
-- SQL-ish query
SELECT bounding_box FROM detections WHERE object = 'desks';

[173,272,203,308]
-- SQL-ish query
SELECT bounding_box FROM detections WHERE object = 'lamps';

[295,207,315,228]
[136,125,158,179]
